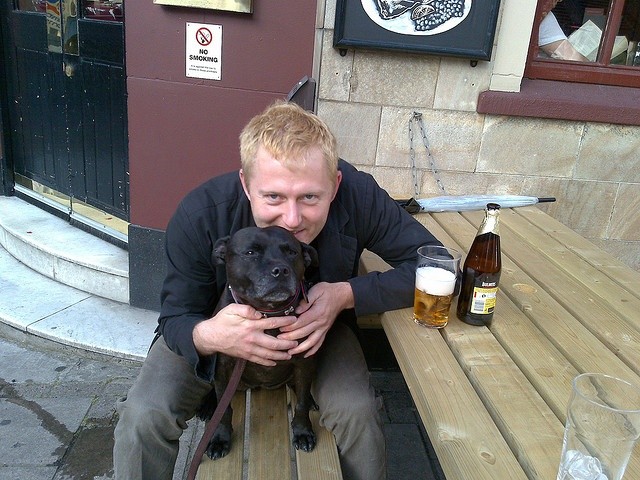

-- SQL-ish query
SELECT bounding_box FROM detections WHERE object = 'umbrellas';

[391,195,557,214]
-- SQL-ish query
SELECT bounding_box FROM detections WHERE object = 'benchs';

[194,368,344,480]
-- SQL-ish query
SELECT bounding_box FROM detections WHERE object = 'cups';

[554,371,640,480]
[412,244,462,330]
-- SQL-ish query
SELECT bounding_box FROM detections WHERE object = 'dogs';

[205,226,320,459]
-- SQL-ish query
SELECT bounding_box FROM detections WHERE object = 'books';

[566,19,629,63]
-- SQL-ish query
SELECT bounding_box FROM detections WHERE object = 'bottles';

[456,203,502,327]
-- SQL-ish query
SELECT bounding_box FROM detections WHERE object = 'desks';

[359,206,640,479]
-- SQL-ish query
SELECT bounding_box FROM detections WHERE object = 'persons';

[110,100,462,480]
[538,0,594,63]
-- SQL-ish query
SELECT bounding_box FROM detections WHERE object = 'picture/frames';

[332,0,501,62]
